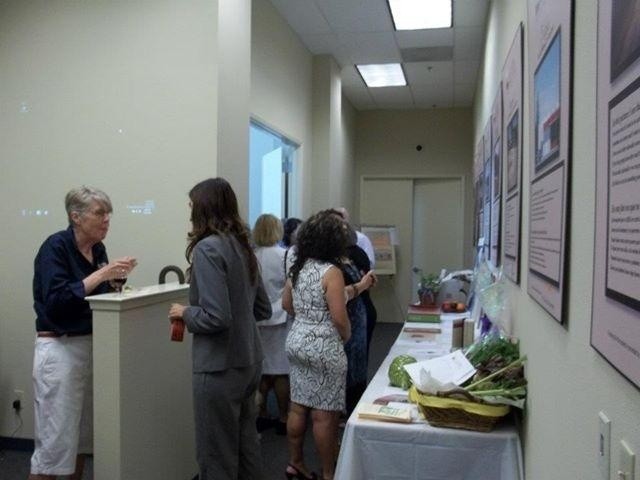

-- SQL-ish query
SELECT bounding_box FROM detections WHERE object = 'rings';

[122,270,126,274]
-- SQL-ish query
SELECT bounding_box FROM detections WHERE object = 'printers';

[418,269,474,306]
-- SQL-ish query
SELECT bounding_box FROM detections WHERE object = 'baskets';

[409,379,512,434]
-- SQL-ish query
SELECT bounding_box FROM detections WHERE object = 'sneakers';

[255,415,287,436]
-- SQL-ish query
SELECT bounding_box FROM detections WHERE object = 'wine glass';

[110,268,128,297]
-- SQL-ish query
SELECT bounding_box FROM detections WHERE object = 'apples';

[456,303,466,312]
[442,303,450,313]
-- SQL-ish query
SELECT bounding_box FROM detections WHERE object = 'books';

[462,319,475,349]
[402,327,443,340]
[357,404,412,423]
[452,318,468,348]
[406,314,441,323]
[409,303,443,315]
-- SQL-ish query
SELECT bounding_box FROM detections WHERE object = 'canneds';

[171,319,184,342]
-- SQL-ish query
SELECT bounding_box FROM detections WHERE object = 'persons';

[248,208,377,478]
[25,183,136,478]
[168,177,272,478]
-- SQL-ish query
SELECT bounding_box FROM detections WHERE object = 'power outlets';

[616,439,636,480]
[12,393,25,412]
[597,412,612,480]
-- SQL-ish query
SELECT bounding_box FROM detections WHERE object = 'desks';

[345,302,519,480]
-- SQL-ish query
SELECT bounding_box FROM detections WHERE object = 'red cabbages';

[388,354,417,389]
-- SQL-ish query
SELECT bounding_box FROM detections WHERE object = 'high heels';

[284,462,321,480]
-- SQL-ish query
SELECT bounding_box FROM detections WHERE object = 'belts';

[35,329,92,338]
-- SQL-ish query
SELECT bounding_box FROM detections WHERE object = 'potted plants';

[411,266,447,305]
